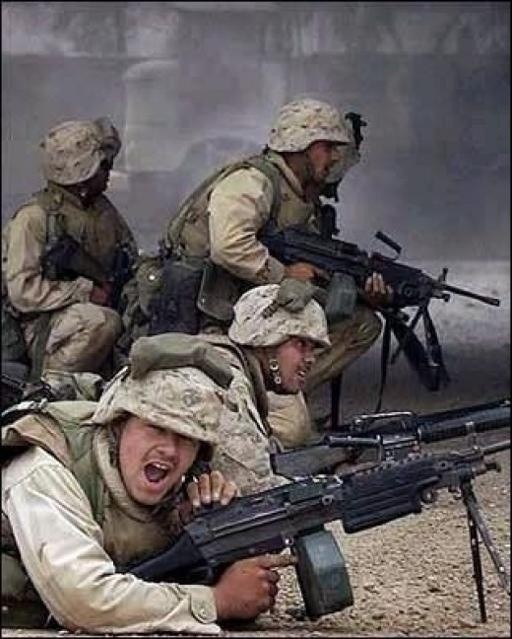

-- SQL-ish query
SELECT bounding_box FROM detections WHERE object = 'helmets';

[227,282,332,350]
[267,96,350,152]
[92,357,224,445]
[39,118,116,185]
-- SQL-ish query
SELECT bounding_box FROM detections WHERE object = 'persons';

[1,332,300,635]
[1,115,139,383]
[199,280,333,498]
[159,98,395,449]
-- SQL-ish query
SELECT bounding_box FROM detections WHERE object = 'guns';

[38,232,123,309]
[270,399,512,478]
[257,229,501,321]
[120,422,511,624]
